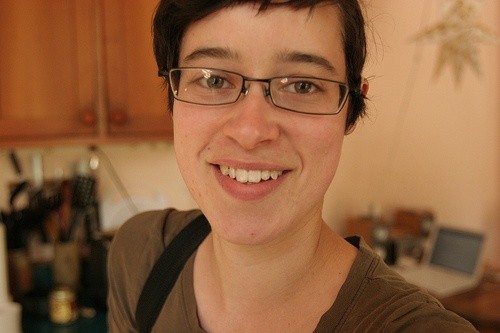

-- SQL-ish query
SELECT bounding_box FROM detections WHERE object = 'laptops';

[399,222,490,299]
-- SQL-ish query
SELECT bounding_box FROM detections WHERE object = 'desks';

[342,233,500,333]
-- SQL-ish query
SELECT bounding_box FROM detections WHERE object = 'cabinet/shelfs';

[0,0,197,141]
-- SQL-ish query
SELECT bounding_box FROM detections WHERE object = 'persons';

[107,0,479,333]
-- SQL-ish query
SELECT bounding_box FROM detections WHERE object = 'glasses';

[161,67,352,116]
